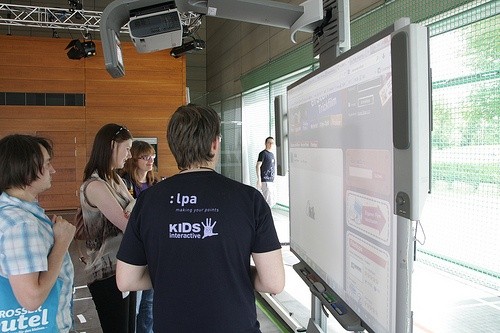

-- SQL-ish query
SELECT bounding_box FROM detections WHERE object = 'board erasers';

[313,282,326,292]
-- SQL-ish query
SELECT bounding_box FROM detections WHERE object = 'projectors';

[128,10,183,53]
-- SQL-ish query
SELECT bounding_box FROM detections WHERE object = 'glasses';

[218,134,222,142]
[117,124,130,136]
[137,153,156,161]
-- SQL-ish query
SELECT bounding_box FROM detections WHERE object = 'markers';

[307,276,315,284]
[323,293,331,302]
[301,269,307,275]
[333,303,343,314]
[326,290,336,301]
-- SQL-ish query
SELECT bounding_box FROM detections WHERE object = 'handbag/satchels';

[0,204,64,333]
[72,206,88,241]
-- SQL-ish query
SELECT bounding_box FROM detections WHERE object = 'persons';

[117,140,156,333]
[0,133,77,333]
[117,101,285,333]
[73,124,137,333]
[256,137,275,209]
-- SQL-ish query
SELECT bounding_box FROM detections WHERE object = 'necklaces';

[177,167,213,173]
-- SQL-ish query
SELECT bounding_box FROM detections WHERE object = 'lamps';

[66,33,98,60]
[169,34,208,60]
